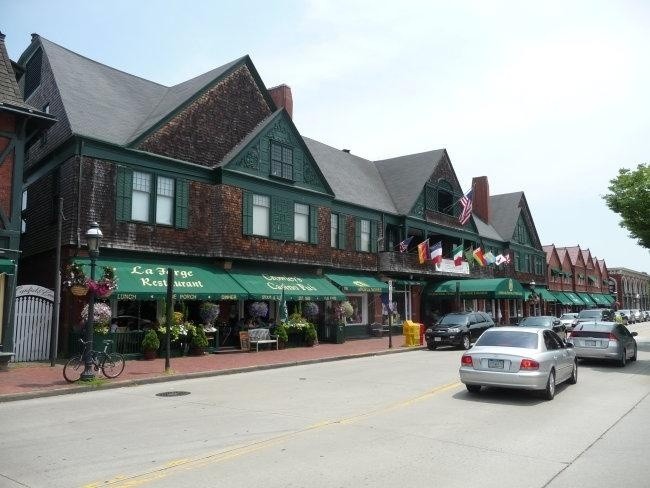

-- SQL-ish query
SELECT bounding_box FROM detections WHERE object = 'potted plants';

[141,329,160,360]
[191,324,209,355]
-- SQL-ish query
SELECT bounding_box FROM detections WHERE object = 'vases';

[97,290,113,298]
[70,285,88,296]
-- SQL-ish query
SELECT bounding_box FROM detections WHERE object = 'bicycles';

[63,339,125,382]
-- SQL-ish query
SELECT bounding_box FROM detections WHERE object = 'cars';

[425,311,494,349]
[459,326,578,400]
[519,307,650,366]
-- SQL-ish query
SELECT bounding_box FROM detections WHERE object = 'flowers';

[303,302,320,318]
[200,302,220,325]
[87,265,120,295]
[62,262,88,287]
[249,302,268,318]
[81,302,112,334]
[331,300,353,318]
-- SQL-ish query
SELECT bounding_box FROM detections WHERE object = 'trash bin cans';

[402,319,424,346]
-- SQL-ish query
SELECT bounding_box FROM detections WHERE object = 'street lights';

[79,220,104,382]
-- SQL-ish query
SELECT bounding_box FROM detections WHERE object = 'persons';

[109,316,121,334]
[244,318,255,328]
[253,317,267,327]
[234,316,245,332]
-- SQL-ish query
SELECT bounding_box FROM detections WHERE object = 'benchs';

[247,329,279,352]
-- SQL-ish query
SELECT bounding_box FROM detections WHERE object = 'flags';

[399,235,415,253]
[503,253,513,265]
[494,251,507,267]
[429,241,443,264]
[458,187,473,226]
[450,243,463,267]
[473,246,488,267]
[464,245,476,268]
[416,239,431,264]
[276,282,289,325]
[483,248,497,266]
